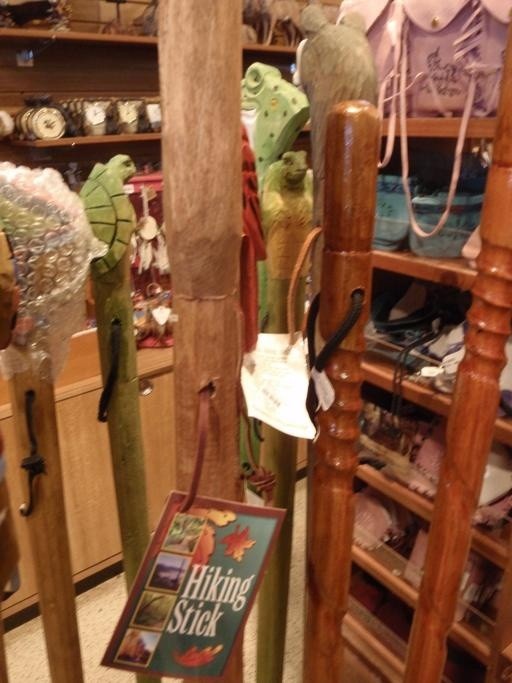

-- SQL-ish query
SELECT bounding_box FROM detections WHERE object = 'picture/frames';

[101,489,286,683]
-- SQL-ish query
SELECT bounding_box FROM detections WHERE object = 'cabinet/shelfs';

[1,35,310,139]
[259,419,308,470]
[301,118,511,683]
[0,325,177,622]
[0,147,174,325]
[0,0,300,48]
